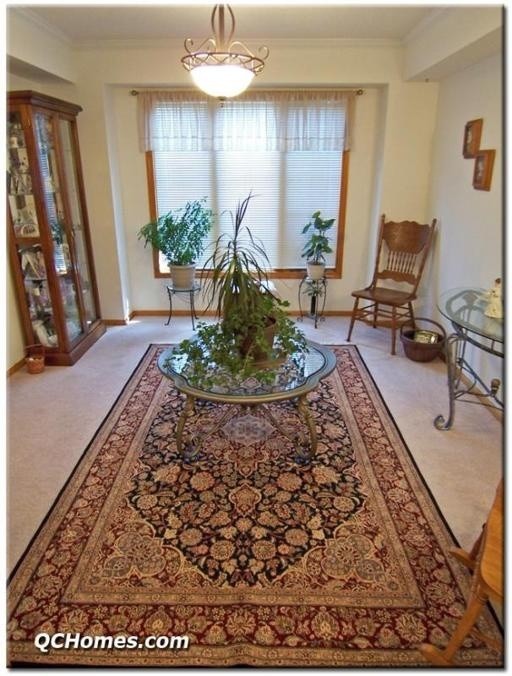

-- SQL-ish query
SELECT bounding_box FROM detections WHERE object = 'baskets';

[23,344,46,373]
[400,317,447,362]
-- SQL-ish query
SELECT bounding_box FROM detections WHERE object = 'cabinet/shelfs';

[8,90,108,367]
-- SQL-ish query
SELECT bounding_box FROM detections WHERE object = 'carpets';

[6,341,503,668]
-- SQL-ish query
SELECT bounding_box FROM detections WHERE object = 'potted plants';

[170,186,308,393]
[136,195,220,290]
[298,210,336,281]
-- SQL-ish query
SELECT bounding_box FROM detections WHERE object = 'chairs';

[346,213,437,356]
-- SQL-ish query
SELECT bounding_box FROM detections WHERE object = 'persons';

[484,277,503,318]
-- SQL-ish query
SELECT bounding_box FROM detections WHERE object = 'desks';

[156,334,337,459]
[432,284,504,432]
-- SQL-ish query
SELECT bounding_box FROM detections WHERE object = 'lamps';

[178,4,270,108]
